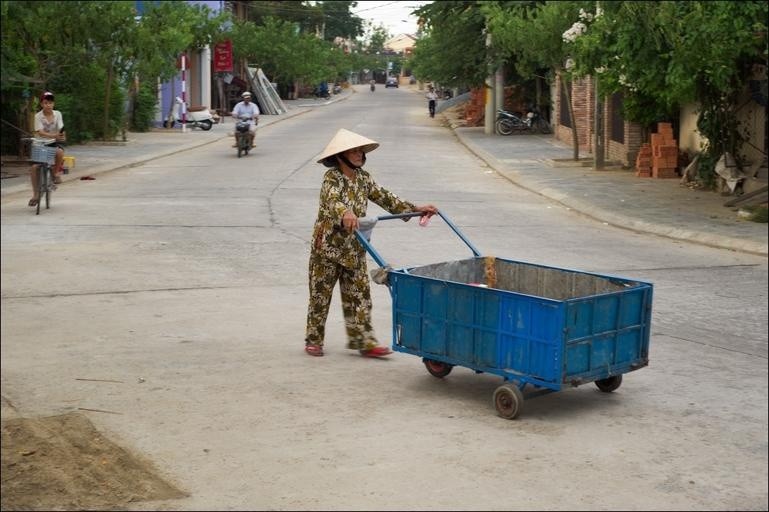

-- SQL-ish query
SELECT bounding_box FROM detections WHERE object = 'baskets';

[30,143,57,165]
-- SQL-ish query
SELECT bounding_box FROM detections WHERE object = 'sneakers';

[232,143,238,147]
[252,143,256,147]
[307,344,324,356]
[359,345,392,356]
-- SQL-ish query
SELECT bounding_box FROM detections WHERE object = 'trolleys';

[334,210,654,418]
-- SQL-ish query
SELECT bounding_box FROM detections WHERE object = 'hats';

[316,129,380,162]
[242,92,252,97]
[40,92,54,102]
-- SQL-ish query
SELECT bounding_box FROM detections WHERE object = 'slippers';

[28,199,37,205]
[53,169,63,183]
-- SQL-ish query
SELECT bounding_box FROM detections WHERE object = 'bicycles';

[22,137,55,215]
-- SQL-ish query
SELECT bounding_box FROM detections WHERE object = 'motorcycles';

[164,96,215,131]
[233,114,253,157]
[312,88,330,99]
[495,107,552,135]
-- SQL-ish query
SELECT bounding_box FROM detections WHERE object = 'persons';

[425,88,439,118]
[28,92,65,206]
[304,128,438,357]
[369,80,375,94]
[232,91,260,147]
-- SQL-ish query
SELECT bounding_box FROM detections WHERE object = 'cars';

[385,77,398,89]
